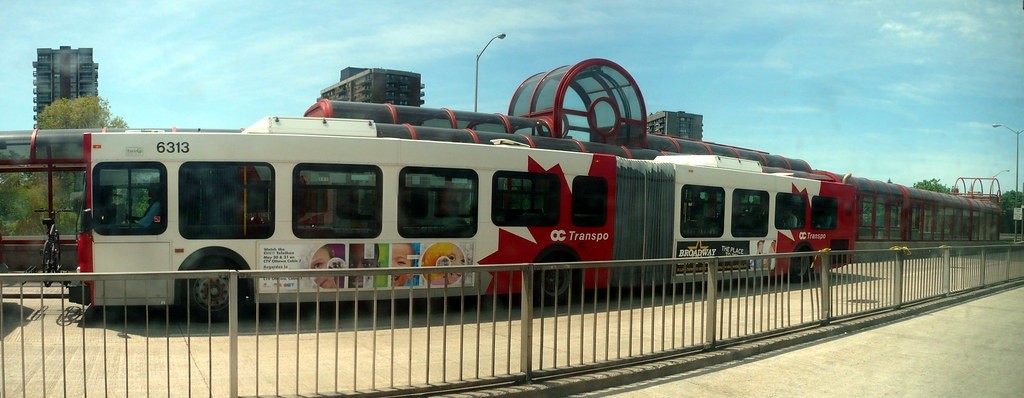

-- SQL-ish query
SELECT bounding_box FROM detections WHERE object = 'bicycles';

[34,208,73,287]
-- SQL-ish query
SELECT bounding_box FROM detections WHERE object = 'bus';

[66,115,859,323]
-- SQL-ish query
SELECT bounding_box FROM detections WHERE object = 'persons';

[349,243,381,288]
[309,244,339,289]
[123,176,163,228]
[750,239,765,270]
[818,206,833,229]
[391,243,415,286]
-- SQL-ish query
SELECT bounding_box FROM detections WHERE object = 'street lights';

[474,32,506,112]
[992,170,1010,198]
[992,123,1024,245]
